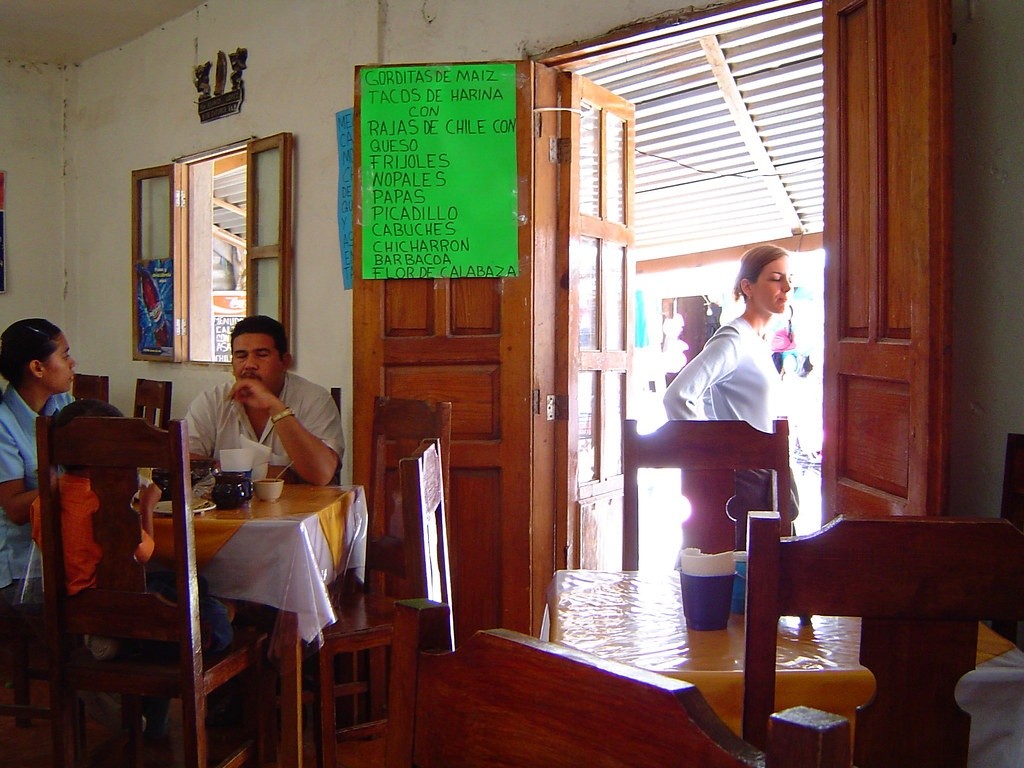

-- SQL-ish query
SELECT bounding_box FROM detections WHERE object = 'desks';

[541,570,1024,768]
[131,484,371,768]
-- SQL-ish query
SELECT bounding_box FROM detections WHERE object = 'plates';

[151,496,216,516]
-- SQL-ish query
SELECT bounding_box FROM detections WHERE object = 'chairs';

[389,597,853,768]
[0,371,453,768]
[743,504,1024,768]
[623,415,791,571]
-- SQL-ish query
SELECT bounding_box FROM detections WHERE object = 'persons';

[664,244,801,567]
[29,400,243,737]
[1,318,76,611]
[154,315,344,716]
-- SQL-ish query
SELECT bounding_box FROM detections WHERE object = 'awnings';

[211,1,825,274]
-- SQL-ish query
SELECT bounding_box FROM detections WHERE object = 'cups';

[729,561,746,613]
[679,570,736,631]
[252,478,284,500]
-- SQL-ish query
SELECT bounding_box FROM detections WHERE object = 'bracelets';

[271,407,295,423]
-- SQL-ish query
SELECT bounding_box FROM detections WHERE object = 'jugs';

[211,470,254,508]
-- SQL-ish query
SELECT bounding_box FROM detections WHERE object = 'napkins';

[681,547,734,574]
[219,449,252,473]
[240,435,273,480]
